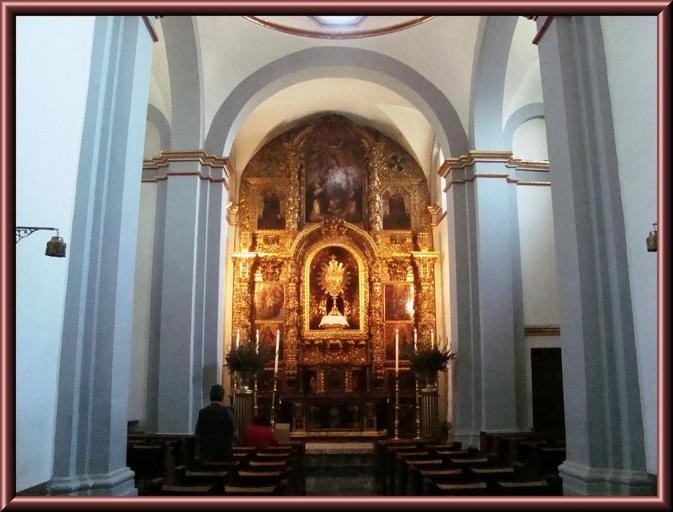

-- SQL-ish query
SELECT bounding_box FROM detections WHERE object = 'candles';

[431,332,435,350]
[395,332,399,372]
[236,330,239,350]
[254,330,259,355]
[414,332,418,351]
[273,330,281,373]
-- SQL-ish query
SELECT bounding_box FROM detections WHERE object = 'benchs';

[290,431,384,477]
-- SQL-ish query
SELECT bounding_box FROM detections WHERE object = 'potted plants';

[222,340,290,390]
[402,338,458,389]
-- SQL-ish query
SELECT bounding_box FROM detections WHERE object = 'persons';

[239,412,280,456]
[194,384,237,460]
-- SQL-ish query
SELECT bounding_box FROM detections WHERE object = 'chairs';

[376,432,565,496]
[128,435,303,496]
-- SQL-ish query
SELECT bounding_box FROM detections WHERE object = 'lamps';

[16,226,66,258]
[645,223,657,250]
